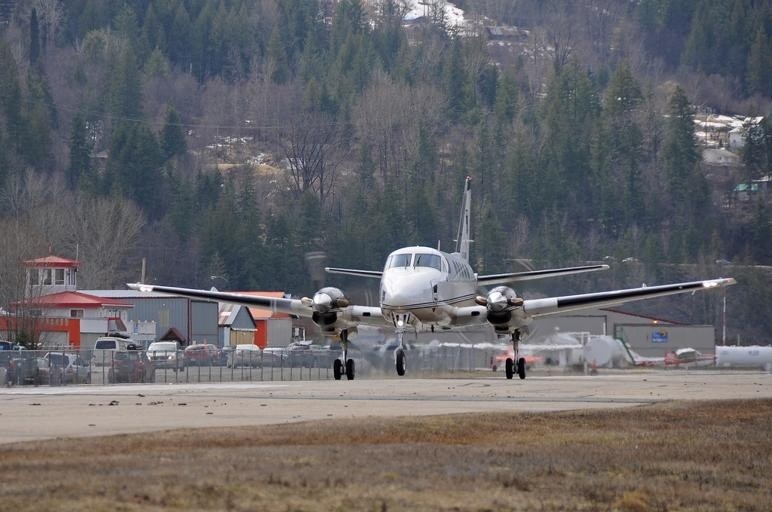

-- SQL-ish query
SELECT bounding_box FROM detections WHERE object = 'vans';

[93,336,137,367]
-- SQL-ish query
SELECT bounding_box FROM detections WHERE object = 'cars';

[227,343,263,369]
[107,349,156,384]
[263,341,331,368]
[0,340,92,387]
[184,344,223,366]
[145,340,185,372]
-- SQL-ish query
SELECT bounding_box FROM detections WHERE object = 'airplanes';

[124,174,739,383]
